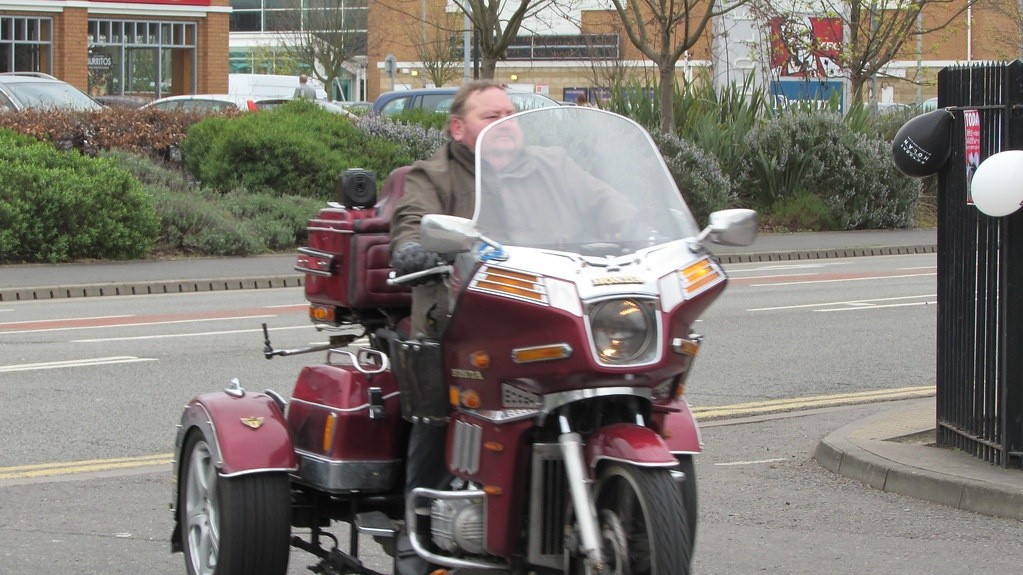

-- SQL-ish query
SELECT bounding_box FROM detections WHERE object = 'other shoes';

[394,533,434,575]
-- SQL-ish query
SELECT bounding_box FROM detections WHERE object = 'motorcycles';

[168,107,760,575]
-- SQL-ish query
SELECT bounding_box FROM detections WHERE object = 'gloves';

[392,242,439,287]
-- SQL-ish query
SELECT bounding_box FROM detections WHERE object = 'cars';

[139,94,361,121]
[861,103,913,113]
[918,98,938,111]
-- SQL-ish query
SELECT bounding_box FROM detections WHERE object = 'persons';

[293,74,317,99]
[390,82,674,574]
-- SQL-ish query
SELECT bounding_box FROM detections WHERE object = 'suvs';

[0,71,110,114]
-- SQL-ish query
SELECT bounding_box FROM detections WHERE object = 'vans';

[372,89,563,117]
